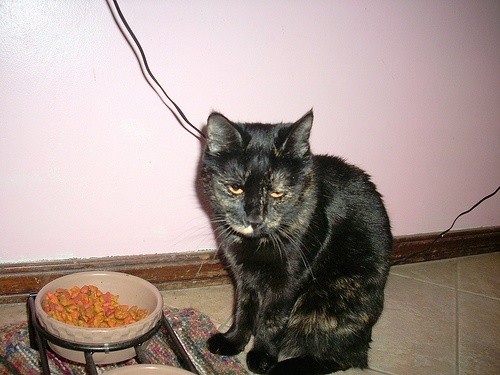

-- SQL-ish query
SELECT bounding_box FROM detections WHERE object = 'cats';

[196,112,393,375]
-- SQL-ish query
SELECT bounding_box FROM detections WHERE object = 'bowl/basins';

[33,271,162,365]
